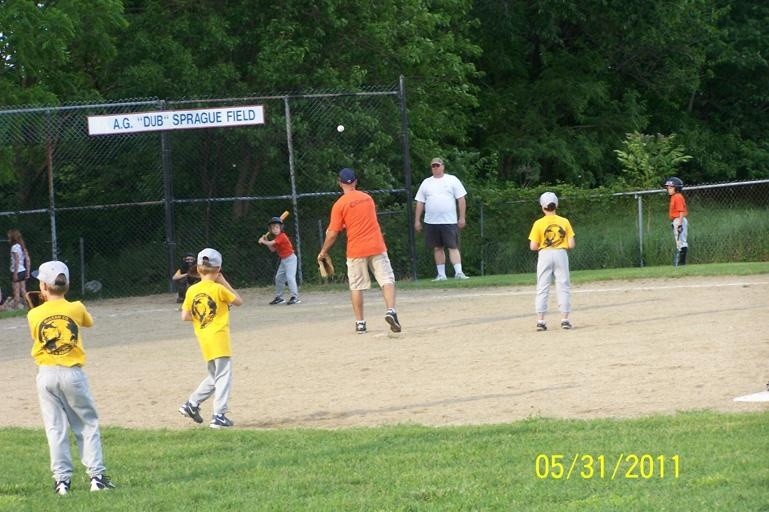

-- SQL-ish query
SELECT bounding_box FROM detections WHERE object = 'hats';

[182,252,197,265]
[431,157,443,167]
[31,260,69,286]
[338,168,355,184]
[540,192,559,210]
[197,248,222,267]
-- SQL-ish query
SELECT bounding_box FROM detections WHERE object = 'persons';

[25,260,116,495]
[317,167,402,334]
[527,190,576,330]
[6,227,31,308]
[413,157,471,281]
[258,216,302,305]
[665,176,688,267]
[178,247,243,427]
[172,252,198,303]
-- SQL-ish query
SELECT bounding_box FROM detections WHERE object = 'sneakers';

[385,311,401,332]
[179,400,204,423]
[287,297,301,305]
[176,296,184,303]
[432,274,447,282]
[536,323,546,331]
[356,321,367,334]
[454,273,470,280]
[561,321,571,328]
[53,479,71,496]
[210,413,234,429]
[269,296,285,304]
[90,474,116,492]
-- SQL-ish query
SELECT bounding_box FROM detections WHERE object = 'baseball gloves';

[187,264,201,278]
[318,254,335,276]
[27,291,45,309]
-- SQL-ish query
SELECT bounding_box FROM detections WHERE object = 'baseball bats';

[263,211,289,238]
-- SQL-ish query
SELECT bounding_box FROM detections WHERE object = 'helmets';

[267,217,284,232]
[665,177,684,188]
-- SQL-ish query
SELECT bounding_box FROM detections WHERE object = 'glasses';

[432,164,440,168]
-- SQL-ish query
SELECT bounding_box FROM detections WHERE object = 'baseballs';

[337,125,344,132]
